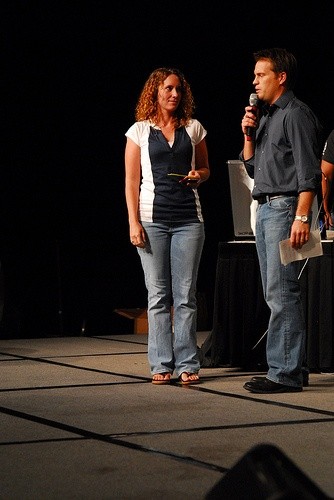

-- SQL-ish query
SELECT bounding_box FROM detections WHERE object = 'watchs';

[294,215,310,224]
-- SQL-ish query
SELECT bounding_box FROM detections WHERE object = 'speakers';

[204,444,334,500]
[228,160,320,238]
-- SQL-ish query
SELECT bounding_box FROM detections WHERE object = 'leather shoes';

[251,376,309,387]
[243,382,303,394]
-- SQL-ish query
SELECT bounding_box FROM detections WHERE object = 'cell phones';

[182,179,200,186]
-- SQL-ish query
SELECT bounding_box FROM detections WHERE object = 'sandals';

[152,372,171,385]
[176,372,200,385]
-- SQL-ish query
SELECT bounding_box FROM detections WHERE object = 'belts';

[258,192,299,204]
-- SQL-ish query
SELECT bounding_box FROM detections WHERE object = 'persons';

[125,67,211,385]
[240,48,334,394]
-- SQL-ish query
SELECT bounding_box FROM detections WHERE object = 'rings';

[131,241,134,243]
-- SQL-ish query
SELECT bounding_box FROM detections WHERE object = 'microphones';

[246,93,258,141]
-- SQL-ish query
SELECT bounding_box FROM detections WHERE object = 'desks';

[198,239,334,372]
